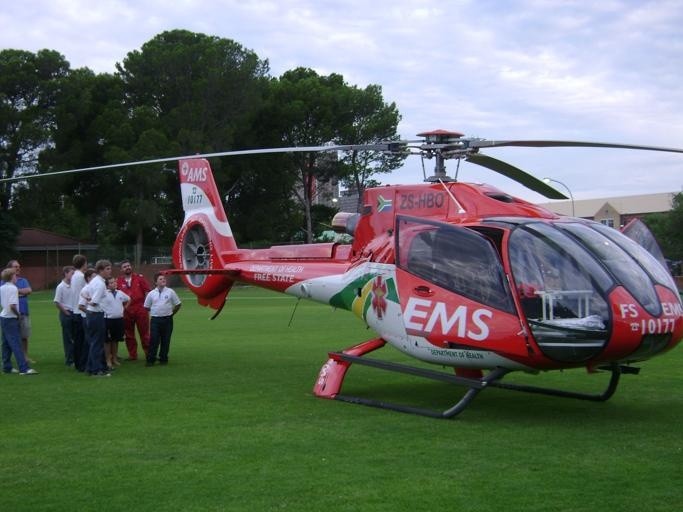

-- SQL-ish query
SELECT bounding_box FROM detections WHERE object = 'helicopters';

[0,89,683,425]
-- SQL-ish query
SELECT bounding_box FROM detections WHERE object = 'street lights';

[539,176,577,220]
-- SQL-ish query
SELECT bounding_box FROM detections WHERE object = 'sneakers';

[92,366,116,377]
[10,368,40,376]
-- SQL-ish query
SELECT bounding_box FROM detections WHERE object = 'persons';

[0,260,39,374]
[53,254,182,377]
